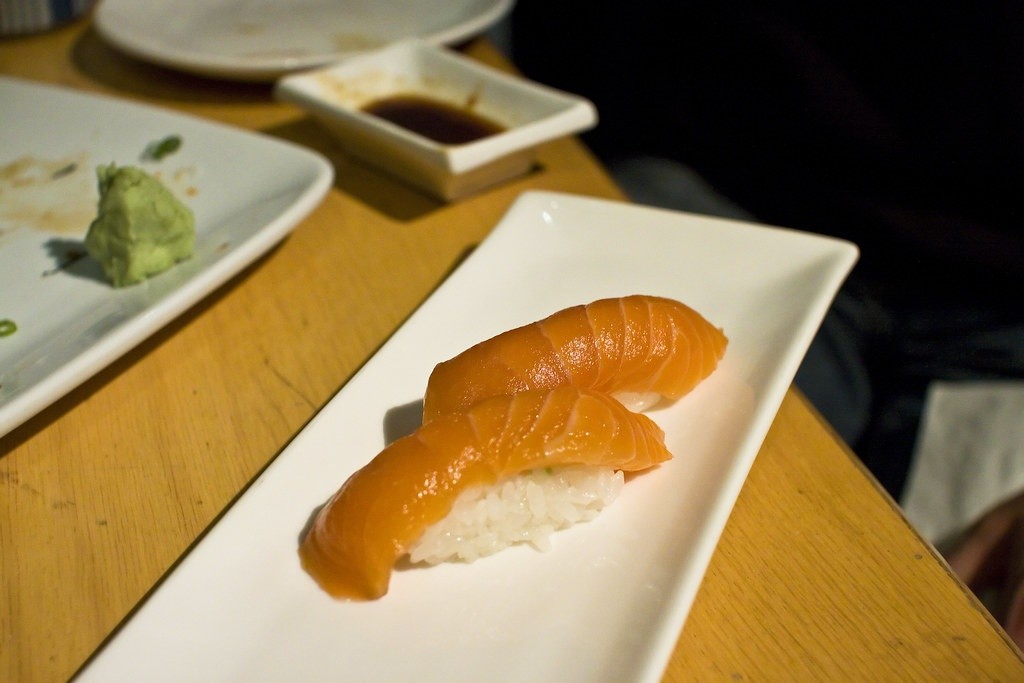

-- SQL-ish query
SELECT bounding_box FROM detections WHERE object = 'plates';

[0,73,335,440]
[68,188,860,683]
[91,0,513,83]
[275,34,598,203]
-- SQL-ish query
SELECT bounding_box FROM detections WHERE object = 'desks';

[0,8,1024,683]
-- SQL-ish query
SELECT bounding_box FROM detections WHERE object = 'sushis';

[307,391,671,602]
[422,294,729,413]
